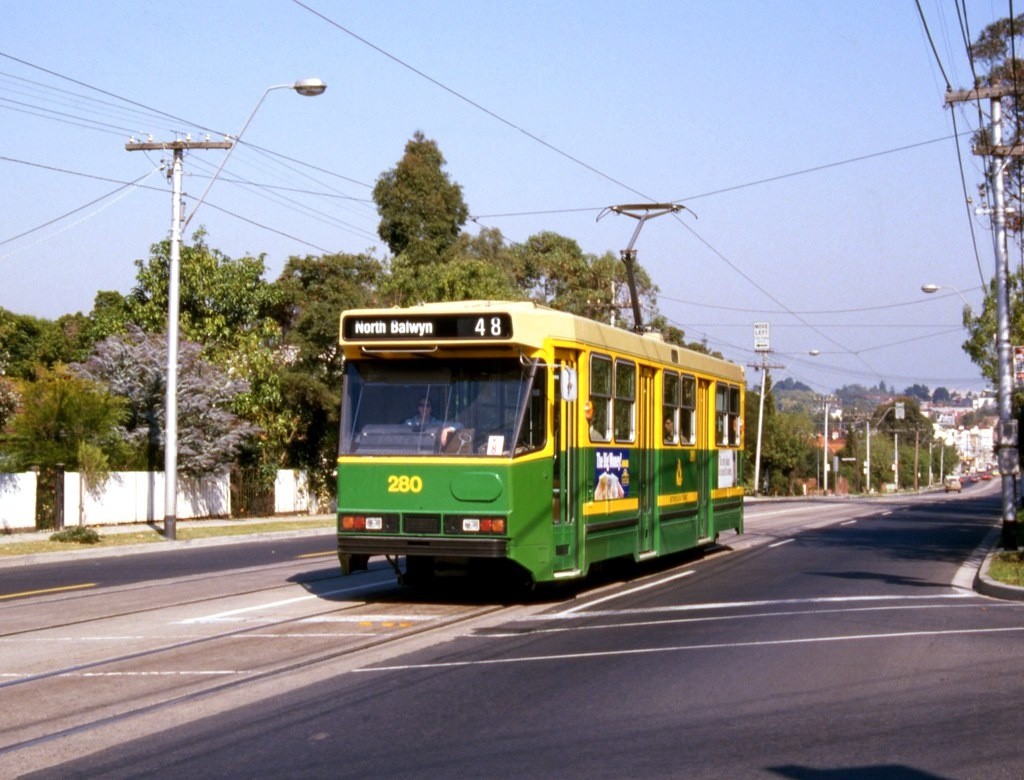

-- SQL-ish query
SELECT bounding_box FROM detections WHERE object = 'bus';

[335,299,746,587]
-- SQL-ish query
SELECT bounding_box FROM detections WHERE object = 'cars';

[944,475,962,493]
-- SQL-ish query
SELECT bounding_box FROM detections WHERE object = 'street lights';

[753,349,819,498]
[124,78,326,539]
[921,283,982,321]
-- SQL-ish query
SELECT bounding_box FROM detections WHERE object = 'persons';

[663,414,688,443]
[402,396,464,447]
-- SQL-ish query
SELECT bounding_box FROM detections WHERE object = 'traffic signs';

[753,321,770,351]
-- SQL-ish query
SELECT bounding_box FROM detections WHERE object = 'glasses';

[418,403,431,408]
